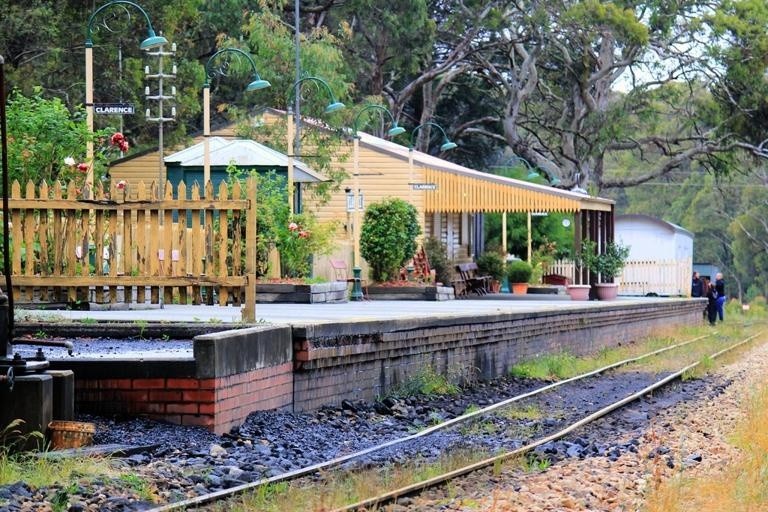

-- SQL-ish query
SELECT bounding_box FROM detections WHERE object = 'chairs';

[330,258,371,302]
[443,263,494,299]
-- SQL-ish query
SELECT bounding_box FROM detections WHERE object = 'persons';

[693,272,726,326]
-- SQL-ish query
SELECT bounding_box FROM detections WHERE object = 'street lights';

[354,102,405,218]
[201,35,272,201]
[407,120,457,222]
[285,72,346,224]
[82,1,172,202]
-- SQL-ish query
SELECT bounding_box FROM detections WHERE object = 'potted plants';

[566,236,632,301]
[476,250,533,295]
